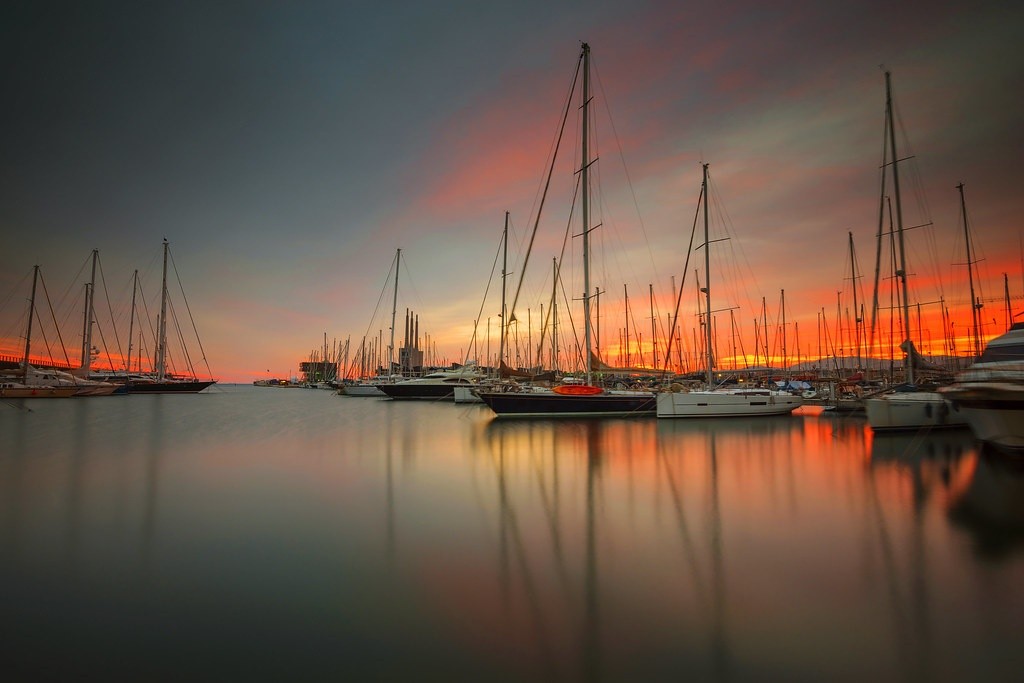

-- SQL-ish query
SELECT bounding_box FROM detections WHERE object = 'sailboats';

[655,159,805,416]
[250,182,1014,416]
[476,40,657,420]
[862,69,972,434]
[0,231,221,399]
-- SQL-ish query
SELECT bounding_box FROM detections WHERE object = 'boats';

[936,320,1024,459]
